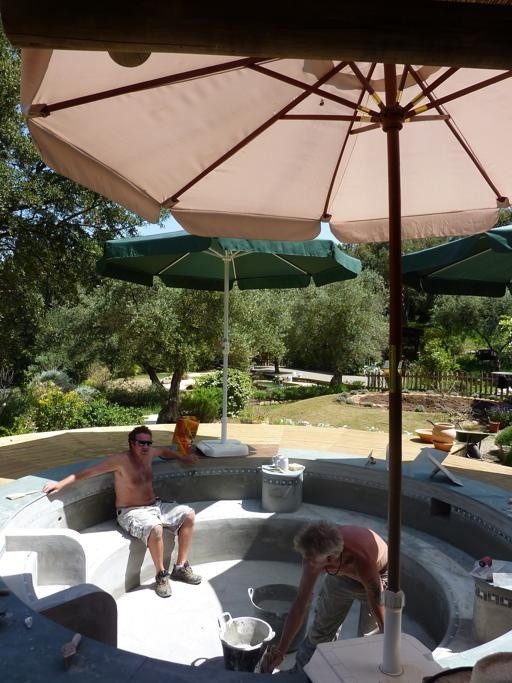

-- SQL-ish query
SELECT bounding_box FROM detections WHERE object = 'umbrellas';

[95,230,362,444]
[400,224,512,297]
[18,44,512,592]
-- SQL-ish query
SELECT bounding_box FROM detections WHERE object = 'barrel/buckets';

[248,586,310,654]
[472,558,511,641]
[260,463,305,512]
[488,419,500,433]
[216,612,277,672]
[432,422,456,451]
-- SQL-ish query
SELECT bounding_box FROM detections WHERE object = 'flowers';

[484,405,503,421]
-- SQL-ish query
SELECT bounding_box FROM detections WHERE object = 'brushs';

[6,490,42,501]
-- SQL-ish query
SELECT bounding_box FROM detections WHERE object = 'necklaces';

[325,553,342,576]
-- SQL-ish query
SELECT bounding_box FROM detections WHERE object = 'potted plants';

[494,426,512,455]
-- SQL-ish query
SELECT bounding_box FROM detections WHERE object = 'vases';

[488,421,500,433]
[382,367,403,386]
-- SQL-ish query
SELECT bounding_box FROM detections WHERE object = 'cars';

[383,360,405,376]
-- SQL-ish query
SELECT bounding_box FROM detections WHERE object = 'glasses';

[136,440,152,445]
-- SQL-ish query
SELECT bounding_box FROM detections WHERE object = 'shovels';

[60,633,82,658]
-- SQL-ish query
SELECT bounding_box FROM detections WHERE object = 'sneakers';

[171,562,202,584]
[155,569,172,598]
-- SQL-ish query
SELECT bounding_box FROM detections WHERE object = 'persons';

[262,520,389,675]
[43,426,202,598]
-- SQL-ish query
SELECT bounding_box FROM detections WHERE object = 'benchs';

[0,455,512,683]
